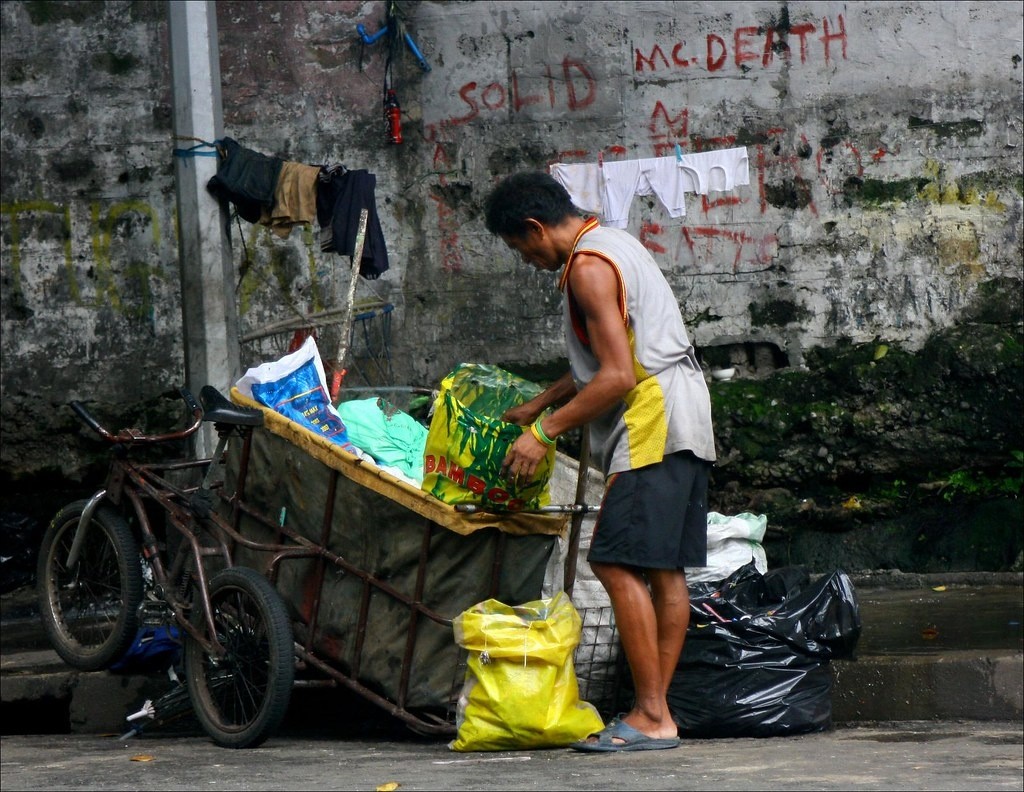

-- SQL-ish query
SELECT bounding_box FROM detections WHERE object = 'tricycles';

[34,383,617,751]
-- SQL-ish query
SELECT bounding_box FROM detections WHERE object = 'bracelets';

[530,420,556,447]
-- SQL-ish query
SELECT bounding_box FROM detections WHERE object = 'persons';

[484,170,715,752]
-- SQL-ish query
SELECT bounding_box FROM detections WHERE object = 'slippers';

[569,713,681,752]
